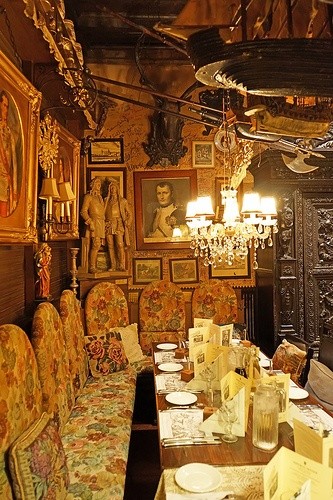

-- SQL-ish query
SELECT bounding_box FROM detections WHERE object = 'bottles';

[233,349,248,379]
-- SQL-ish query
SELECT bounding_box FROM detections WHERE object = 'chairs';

[272,333,333,411]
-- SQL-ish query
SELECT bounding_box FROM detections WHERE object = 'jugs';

[252,379,280,453]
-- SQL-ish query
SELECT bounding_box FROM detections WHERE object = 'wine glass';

[177,333,294,444]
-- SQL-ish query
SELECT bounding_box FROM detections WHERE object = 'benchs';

[0,281,238,500]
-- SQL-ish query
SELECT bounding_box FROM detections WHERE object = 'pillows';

[272,339,307,383]
[84,323,147,375]
[9,412,71,500]
[303,359,333,413]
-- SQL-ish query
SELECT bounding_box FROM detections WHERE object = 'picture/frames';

[88,138,124,163]
[133,258,163,285]
[209,247,252,278]
[133,170,198,249]
[0,51,80,243]
[86,167,127,199]
[192,140,215,168]
[169,258,199,284]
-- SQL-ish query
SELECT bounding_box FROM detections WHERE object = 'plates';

[232,339,242,346]
[288,388,309,400]
[158,363,184,372]
[175,462,223,493]
[156,342,177,351]
[166,392,197,406]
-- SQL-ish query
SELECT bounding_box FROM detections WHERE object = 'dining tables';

[151,341,333,500]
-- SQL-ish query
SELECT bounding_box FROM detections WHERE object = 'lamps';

[36,175,76,242]
[186,86,278,267]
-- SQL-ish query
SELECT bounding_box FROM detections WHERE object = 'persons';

[103,181,132,272]
[146,182,189,239]
[79,177,106,274]
[0,89,17,219]
